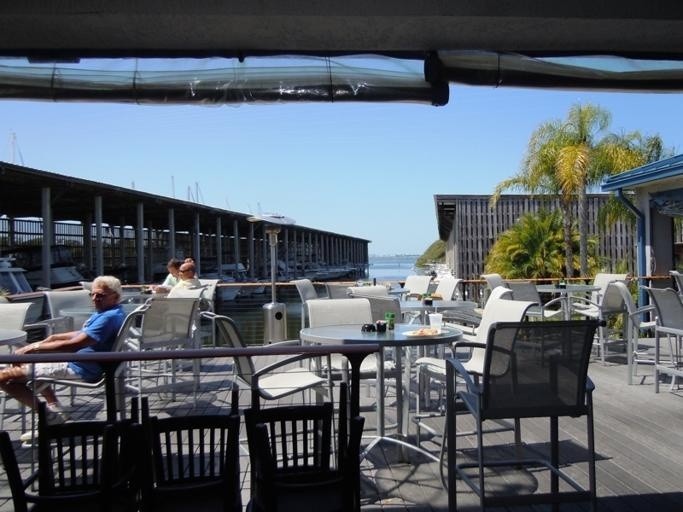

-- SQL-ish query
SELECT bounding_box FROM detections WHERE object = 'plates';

[402,330,450,337]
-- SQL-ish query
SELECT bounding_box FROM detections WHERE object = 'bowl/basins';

[361,324,376,331]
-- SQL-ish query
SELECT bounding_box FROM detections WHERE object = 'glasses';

[89,291,117,298]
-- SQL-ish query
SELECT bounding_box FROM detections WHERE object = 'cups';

[428,313,443,330]
[384,312,395,330]
[377,323,387,332]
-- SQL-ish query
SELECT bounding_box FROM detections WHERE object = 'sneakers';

[20,411,65,443]
[49,405,72,422]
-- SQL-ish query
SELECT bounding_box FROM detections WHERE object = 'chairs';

[124,297,199,410]
[18,303,152,463]
[0,303,36,330]
[43,290,94,318]
[0,417,242,512]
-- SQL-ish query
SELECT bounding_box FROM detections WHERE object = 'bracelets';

[34,341,40,353]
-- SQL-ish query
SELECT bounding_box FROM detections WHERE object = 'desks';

[0,329,28,353]
[59,303,141,318]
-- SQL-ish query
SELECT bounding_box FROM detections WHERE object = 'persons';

[167,258,201,298]
[0,276,127,442]
[149,259,182,292]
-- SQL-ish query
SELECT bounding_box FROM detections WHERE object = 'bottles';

[418,291,432,303]
